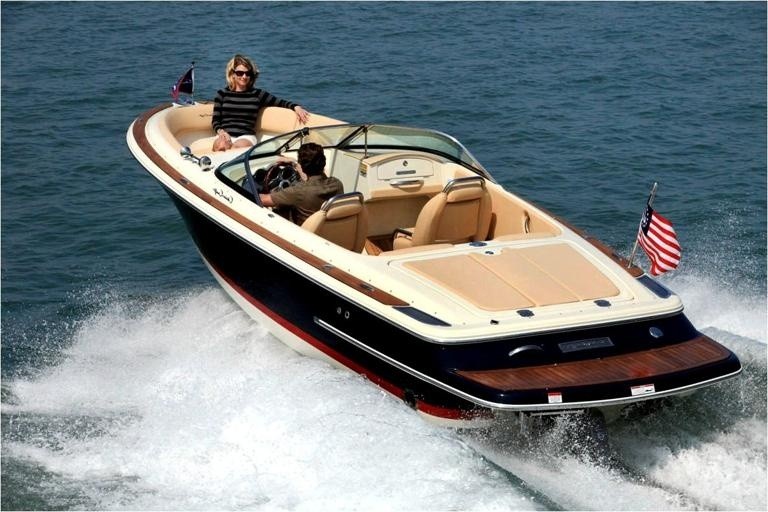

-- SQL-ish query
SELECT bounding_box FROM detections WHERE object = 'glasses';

[233,70,252,76]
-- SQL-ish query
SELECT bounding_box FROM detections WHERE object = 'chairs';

[392,177,494,248]
[297,192,368,255]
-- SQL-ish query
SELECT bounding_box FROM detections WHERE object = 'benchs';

[176,126,275,157]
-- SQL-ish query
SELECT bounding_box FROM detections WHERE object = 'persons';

[210,53,311,152]
[258,142,344,227]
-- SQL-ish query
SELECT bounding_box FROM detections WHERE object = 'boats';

[125,102,742,445]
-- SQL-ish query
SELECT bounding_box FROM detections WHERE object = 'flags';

[171,66,194,103]
[637,195,683,279]
